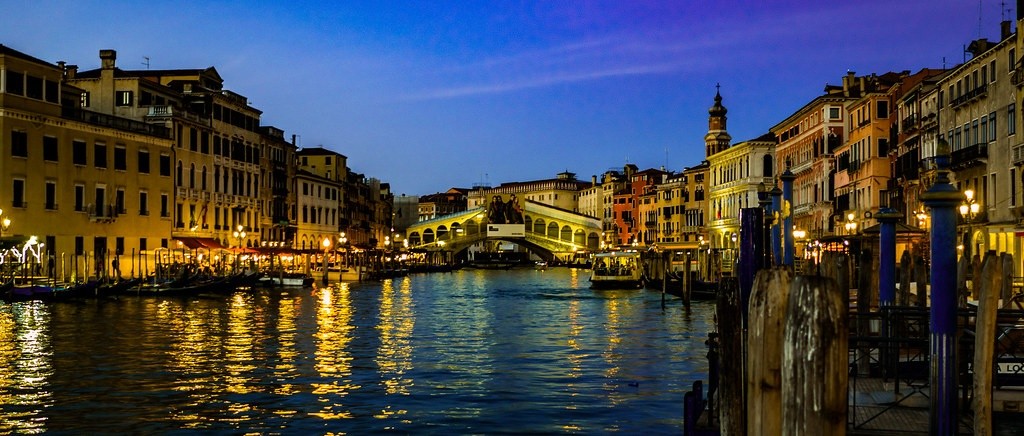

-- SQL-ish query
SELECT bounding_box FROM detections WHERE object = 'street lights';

[960,190,980,272]
[233,224,245,248]
[535,261,548,270]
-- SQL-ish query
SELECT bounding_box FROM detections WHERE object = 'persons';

[596,259,634,277]
[192,257,269,273]
[47,256,54,277]
[36,260,41,275]
[487,194,524,224]
[112,257,120,278]
[643,261,649,279]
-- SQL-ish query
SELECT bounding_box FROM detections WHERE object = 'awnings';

[174,237,324,255]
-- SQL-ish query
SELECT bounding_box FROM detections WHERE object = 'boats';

[575,246,645,288]
[0,266,315,299]
